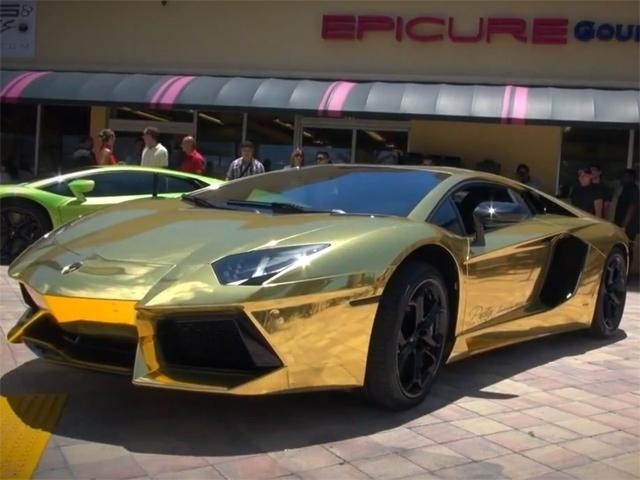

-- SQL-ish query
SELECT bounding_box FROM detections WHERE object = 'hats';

[577,166,592,176]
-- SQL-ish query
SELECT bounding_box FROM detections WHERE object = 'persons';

[282,146,305,169]
[570,165,607,219]
[375,139,404,165]
[517,164,541,188]
[175,135,207,175]
[610,166,640,283]
[141,126,170,168]
[421,154,435,167]
[224,140,265,181]
[314,150,334,165]
[124,139,144,166]
[95,128,119,166]
[60,134,97,173]
[589,162,612,221]
